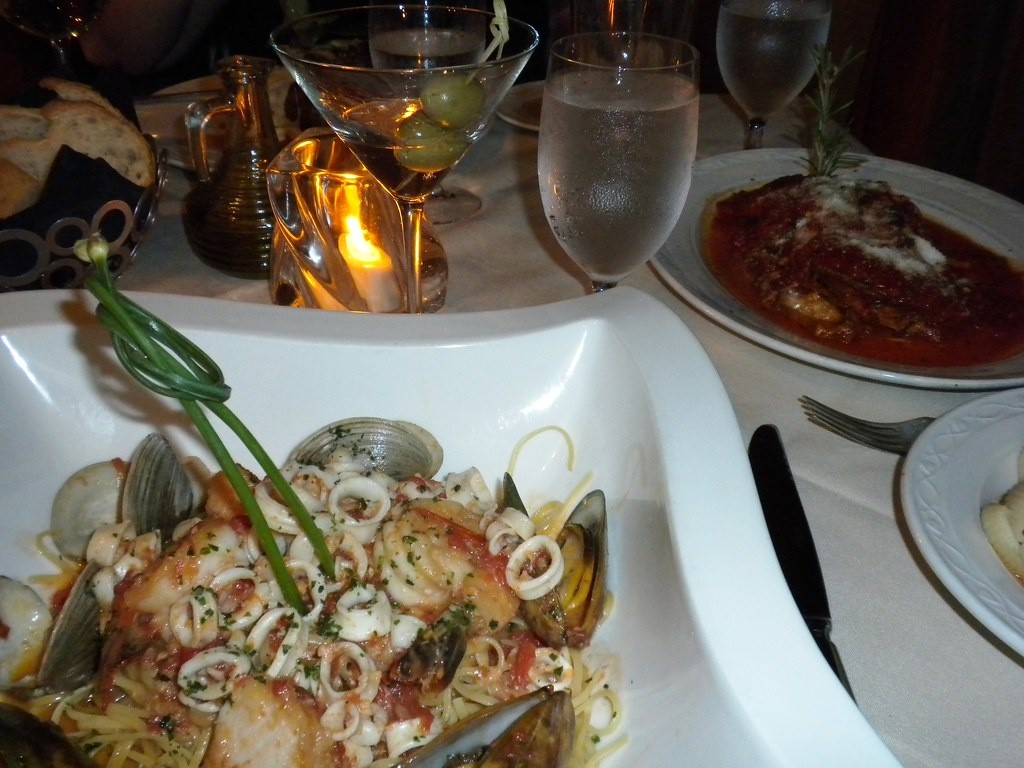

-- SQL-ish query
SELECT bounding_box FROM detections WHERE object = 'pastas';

[13,424,629,767]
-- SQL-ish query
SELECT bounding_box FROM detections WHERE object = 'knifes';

[748,424,858,710]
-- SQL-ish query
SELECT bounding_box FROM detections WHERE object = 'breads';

[0,77,156,222]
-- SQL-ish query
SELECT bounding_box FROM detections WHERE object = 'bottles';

[182,54,290,280]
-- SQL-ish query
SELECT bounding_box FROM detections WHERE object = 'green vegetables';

[791,34,873,176]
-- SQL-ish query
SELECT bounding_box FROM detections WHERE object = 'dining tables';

[0,92,1024,768]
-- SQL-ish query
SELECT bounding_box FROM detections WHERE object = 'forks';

[799,395,936,457]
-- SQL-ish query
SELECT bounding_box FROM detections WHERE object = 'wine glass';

[716,0,832,149]
[269,5,539,314]
[367,0,487,225]
[0,0,101,89]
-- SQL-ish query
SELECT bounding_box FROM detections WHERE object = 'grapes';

[392,70,487,174]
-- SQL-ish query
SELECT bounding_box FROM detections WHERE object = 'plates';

[649,147,1024,389]
[133,94,302,171]
[900,387,1024,661]
[494,78,543,131]
[0,284,907,768]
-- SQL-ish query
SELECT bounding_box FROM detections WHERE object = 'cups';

[569,0,648,70]
[536,29,699,294]
